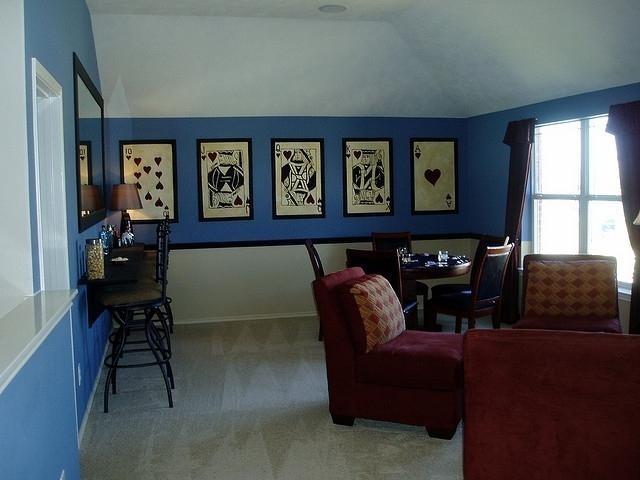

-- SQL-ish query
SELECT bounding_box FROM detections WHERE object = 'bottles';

[99,224,122,257]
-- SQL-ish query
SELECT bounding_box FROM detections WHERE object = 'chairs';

[103,217,177,413]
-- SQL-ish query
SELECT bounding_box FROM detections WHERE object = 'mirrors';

[72,53,107,235]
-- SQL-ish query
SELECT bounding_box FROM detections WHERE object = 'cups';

[438,249,448,262]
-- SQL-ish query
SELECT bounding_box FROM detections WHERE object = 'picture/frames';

[410,137,458,216]
[78,139,93,186]
[198,137,253,221]
[343,137,393,216]
[272,137,325,219]
[119,139,179,224]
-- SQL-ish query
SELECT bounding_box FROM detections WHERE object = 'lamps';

[109,183,147,247]
[80,185,103,220]
